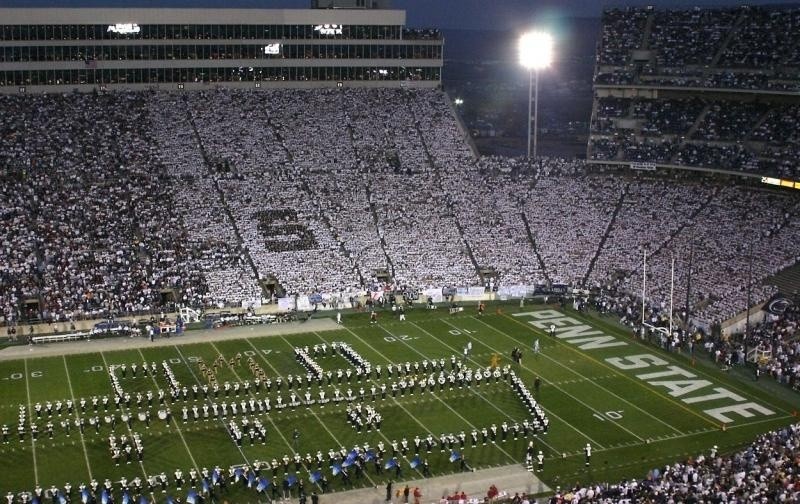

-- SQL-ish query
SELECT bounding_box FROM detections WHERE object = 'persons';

[2,342,490,504]
[492,420,800,504]
[0,84,491,341]
[491,151,800,391]
[584,1,800,154]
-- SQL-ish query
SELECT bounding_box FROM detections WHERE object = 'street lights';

[518,31,553,155]
